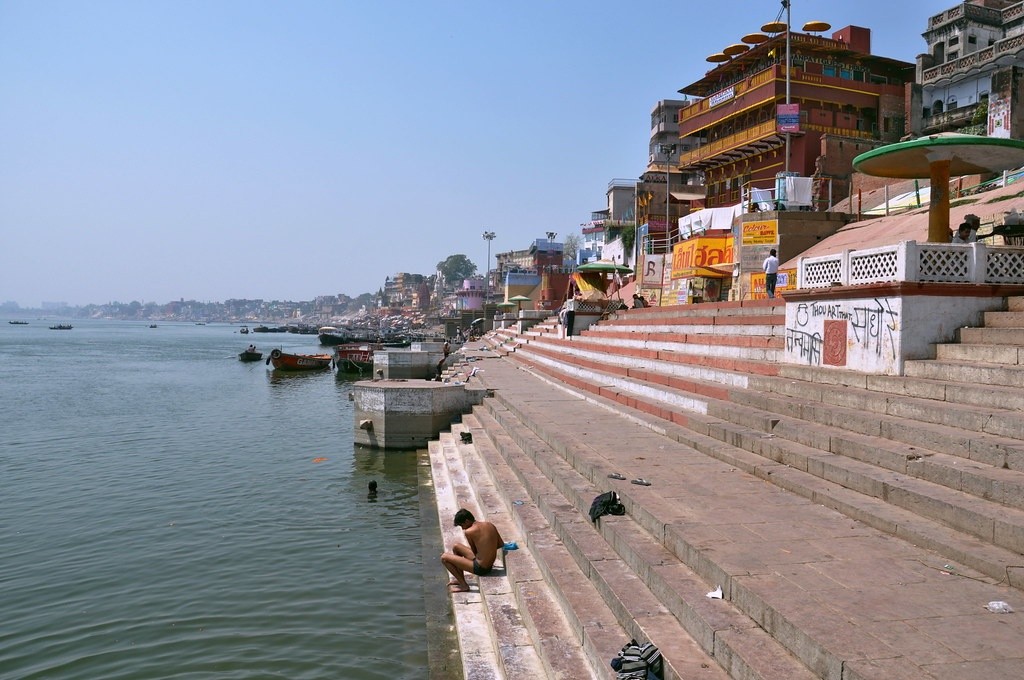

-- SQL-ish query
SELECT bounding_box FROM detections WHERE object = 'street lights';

[482,231,496,304]
[657,142,677,306]
[546,232,558,286]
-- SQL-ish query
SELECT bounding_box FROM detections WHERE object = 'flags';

[618,191,653,226]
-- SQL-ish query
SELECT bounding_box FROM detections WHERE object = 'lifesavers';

[271,349,281,359]
[265,355,271,365]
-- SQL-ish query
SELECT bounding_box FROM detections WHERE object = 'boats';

[337,343,387,374]
[269,345,332,371]
[195,323,205,325]
[241,329,249,334]
[238,351,263,362]
[253,324,318,335]
[150,324,157,328]
[318,326,352,345]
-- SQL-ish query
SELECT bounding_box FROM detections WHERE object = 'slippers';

[631,477,651,486]
[607,472,627,480]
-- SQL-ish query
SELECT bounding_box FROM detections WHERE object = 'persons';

[435,374,442,381]
[762,249,779,299]
[441,508,504,592]
[437,361,442,376]
[949,214,981,244]
[248,344,256,353]
[614,269,624,289]
[443,342,450,358]
[633,294,648,308]
